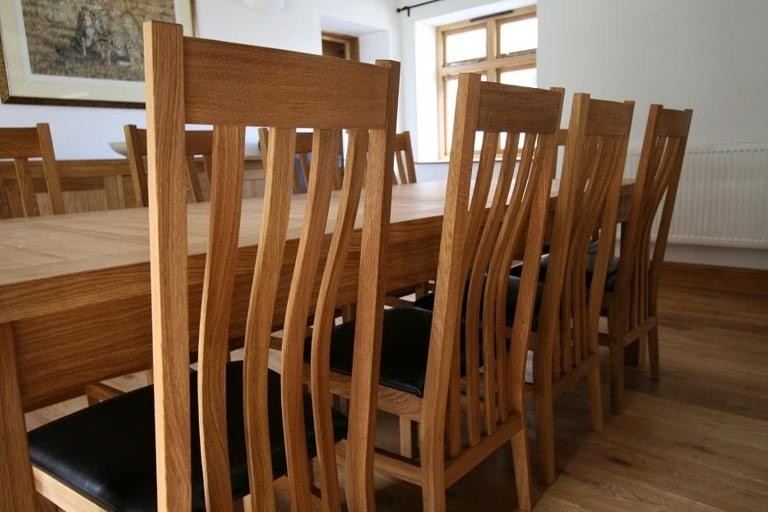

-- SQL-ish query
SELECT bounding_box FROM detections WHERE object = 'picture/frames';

[1,0,200,110]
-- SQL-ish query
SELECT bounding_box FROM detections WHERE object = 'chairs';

[258,128,344,192]
[362,132,418,185]
[510,105,693,417]
[0,122,67,219]
[304,72,564,512]
[412,93,636,486]
[125,125,213,206]
[26,21,401,512]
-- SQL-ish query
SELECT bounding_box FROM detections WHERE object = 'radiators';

[604,143,767,254]
